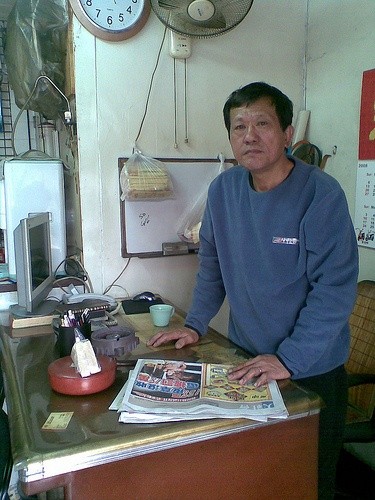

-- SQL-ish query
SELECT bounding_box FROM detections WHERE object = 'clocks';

[68,0,151,42]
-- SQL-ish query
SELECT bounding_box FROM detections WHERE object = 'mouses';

[133,292,156,302]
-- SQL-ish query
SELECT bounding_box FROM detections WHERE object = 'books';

[61,299,117,327]
[10,324,55,336]
[9,304,64,328]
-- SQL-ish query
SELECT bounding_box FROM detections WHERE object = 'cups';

[149,305,175,327]
[52,318,92,358]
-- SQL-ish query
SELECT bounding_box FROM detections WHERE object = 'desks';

[0,294,326,500]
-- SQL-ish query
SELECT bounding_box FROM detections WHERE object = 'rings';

[258,367,261,372]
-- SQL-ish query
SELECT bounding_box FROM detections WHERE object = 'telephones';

[62,283,118,310]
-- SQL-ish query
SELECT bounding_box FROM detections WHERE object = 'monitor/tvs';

[12,211,57,317]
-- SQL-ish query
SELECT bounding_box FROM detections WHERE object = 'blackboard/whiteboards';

[118,157,238,258]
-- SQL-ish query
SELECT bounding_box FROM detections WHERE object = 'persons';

[148,363,186,373]
[148,83,360,500]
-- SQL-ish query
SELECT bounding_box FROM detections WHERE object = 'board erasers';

[162,243,189,256]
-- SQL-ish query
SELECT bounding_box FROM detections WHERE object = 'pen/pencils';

[61,308,91,327]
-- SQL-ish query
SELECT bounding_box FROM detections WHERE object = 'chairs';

[341,279,375,444]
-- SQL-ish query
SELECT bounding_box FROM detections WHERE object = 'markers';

[189,248,199,254]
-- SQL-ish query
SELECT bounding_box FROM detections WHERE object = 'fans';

[150,0,254,58]
